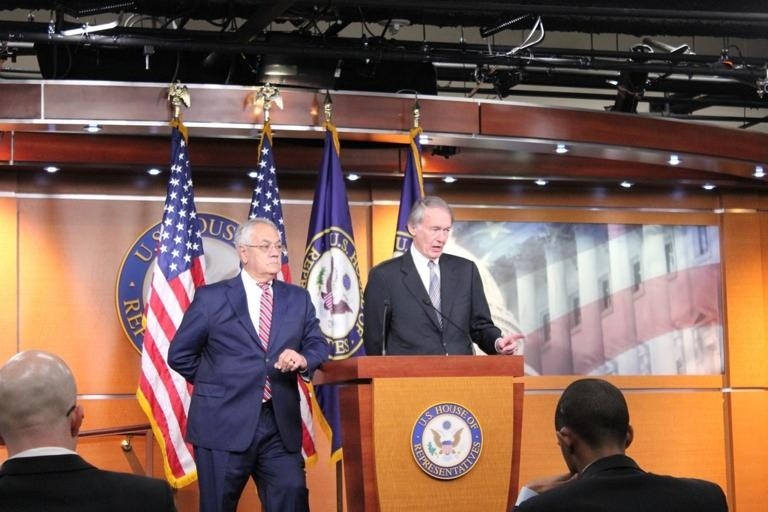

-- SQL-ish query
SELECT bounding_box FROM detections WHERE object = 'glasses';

[246,242,282,252]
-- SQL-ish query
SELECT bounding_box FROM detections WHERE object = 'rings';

[290,359,295,364]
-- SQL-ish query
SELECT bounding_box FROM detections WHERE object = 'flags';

[246,122,318,470]
[135,122,209,489]
[392,126,426,259]
[310,125,366,463]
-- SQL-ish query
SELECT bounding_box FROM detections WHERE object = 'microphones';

[422,297,476,356]
[382,299,391,356]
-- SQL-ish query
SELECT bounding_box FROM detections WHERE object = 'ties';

[256,282,273,403]
[427,262,442,328]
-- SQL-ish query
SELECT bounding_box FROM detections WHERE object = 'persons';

[363,195,527,355]
[0,349,179,512]
[167,217,331,512]
[511,378,730,512]
